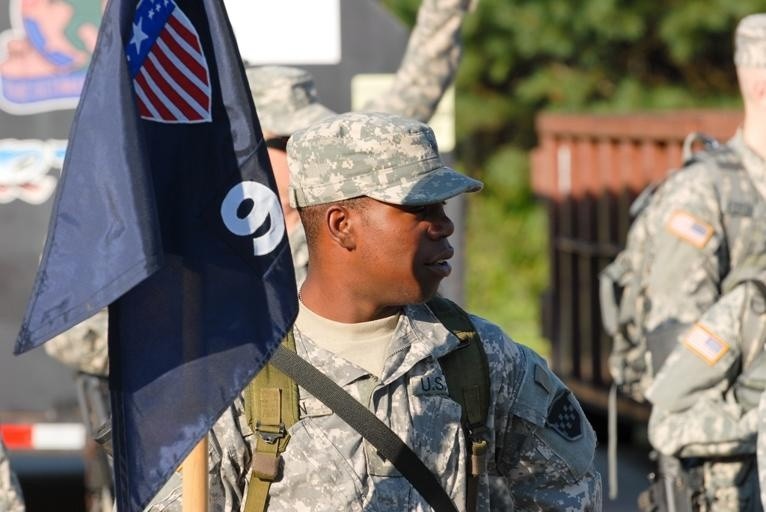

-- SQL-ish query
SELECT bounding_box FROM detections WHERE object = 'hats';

[286,113,484,209]
[733,13,766,65]
[243,66,335,143]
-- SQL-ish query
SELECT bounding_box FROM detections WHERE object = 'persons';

[627,13,766,394]
[42,1,476,377]
[142,111,605,512]
[646,250,766,511]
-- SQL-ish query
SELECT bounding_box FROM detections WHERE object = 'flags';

[12,0,300,512]
